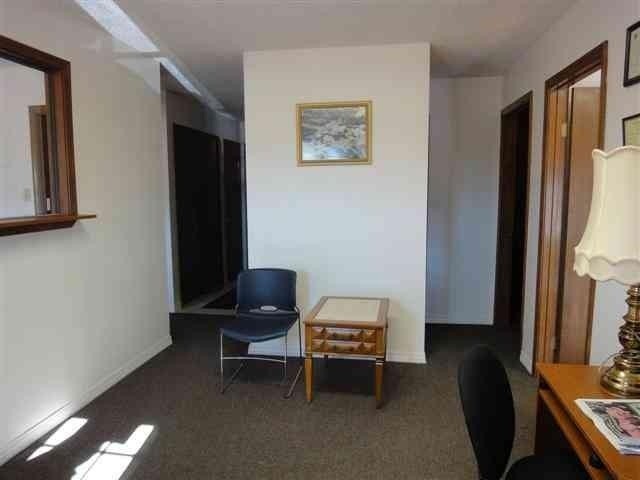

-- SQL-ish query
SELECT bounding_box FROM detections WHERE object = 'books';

[573,396,640,457]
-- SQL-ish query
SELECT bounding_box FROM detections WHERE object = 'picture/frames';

[622,20,640,146]
[295,101,372,167]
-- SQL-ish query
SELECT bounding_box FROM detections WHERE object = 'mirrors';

[0,34,97,236]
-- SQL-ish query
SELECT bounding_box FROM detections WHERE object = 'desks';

[303,295,390,409]
[533,362,640,480]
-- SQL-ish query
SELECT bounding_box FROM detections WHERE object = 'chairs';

[457,345,592,480]
[218,268,304,399]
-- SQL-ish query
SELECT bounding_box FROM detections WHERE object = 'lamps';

[572,145,640,398]
